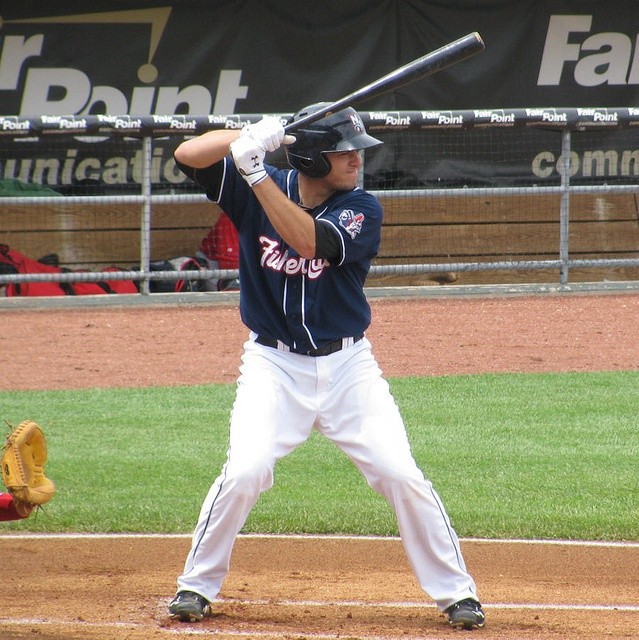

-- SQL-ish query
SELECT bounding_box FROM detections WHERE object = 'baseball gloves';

[413,271,459,286]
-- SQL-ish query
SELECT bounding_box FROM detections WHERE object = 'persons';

[164,100,487,632]
[194,211,240,291]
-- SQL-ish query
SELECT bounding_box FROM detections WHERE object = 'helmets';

[282,101,384,179]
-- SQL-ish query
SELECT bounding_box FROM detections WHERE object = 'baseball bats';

[228,31,487,163]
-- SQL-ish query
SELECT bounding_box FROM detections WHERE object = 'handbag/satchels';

[129,255,209,293]
[1,244,138,296]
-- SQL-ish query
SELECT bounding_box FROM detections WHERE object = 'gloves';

[245,117,297,153]
[230,135,268,187]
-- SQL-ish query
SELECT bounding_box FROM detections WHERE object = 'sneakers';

[167,590,211,621]
[444,597,485,629]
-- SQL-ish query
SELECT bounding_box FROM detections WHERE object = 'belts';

[252,332,365,356]
